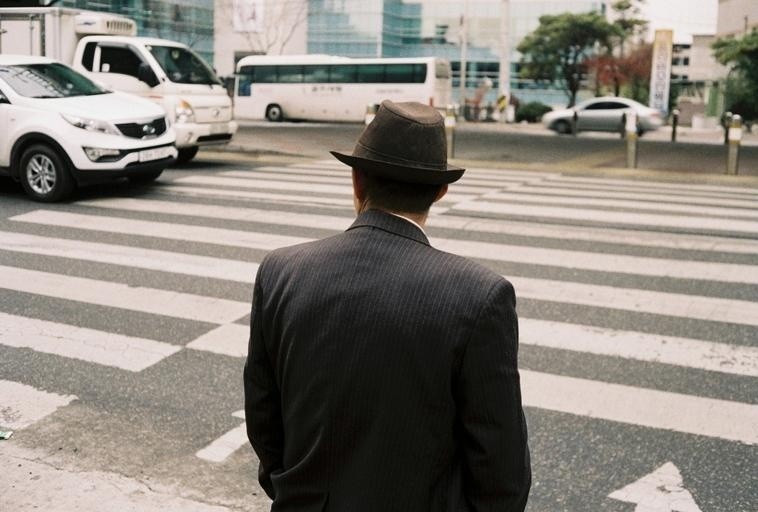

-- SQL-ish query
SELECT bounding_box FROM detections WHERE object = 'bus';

[231,51,454,123]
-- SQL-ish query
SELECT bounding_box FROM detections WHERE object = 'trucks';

[0,6,239,165]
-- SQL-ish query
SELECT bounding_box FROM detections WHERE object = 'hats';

[329,99,466,185]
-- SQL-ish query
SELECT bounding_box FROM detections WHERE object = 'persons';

[239,96,533,512]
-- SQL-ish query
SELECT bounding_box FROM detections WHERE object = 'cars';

[540,96,664,137]
[0,54,178,202]
